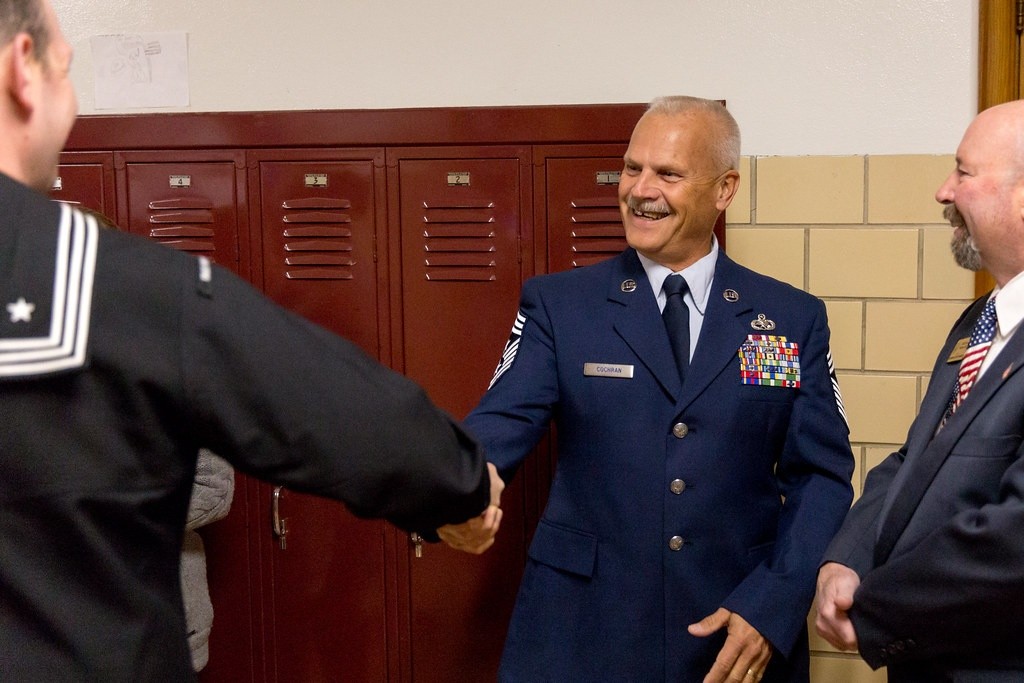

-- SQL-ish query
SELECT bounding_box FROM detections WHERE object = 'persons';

[0,0,505,683]
[807,99,1024,683]
[454,96,855,683]
[181,450,235,683]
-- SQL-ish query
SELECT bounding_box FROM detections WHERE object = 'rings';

[747,669,757,678]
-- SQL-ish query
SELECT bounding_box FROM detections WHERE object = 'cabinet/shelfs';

[48,98,733,683]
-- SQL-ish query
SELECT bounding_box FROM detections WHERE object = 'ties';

[661,275,690,386]
[938,296,999,431]
